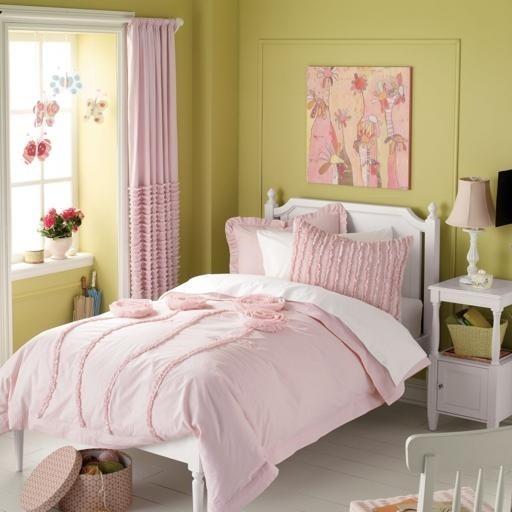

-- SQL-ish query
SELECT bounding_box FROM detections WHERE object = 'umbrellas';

[73,270,104,321]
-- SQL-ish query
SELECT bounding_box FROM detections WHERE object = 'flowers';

[37,206,85,240]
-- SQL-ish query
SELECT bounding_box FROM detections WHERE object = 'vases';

[47,237,72,261]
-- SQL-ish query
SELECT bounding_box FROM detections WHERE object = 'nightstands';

[426,272,511,431]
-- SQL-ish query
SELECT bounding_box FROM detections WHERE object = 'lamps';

[444,176,497,287]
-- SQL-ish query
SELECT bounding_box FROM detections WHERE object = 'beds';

[2,187,440,512]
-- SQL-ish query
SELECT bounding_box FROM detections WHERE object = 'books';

[454,307,492,327]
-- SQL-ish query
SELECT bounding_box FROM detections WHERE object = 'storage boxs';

[20,446,134,512]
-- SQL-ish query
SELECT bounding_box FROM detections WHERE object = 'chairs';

[348,424,512,512]
